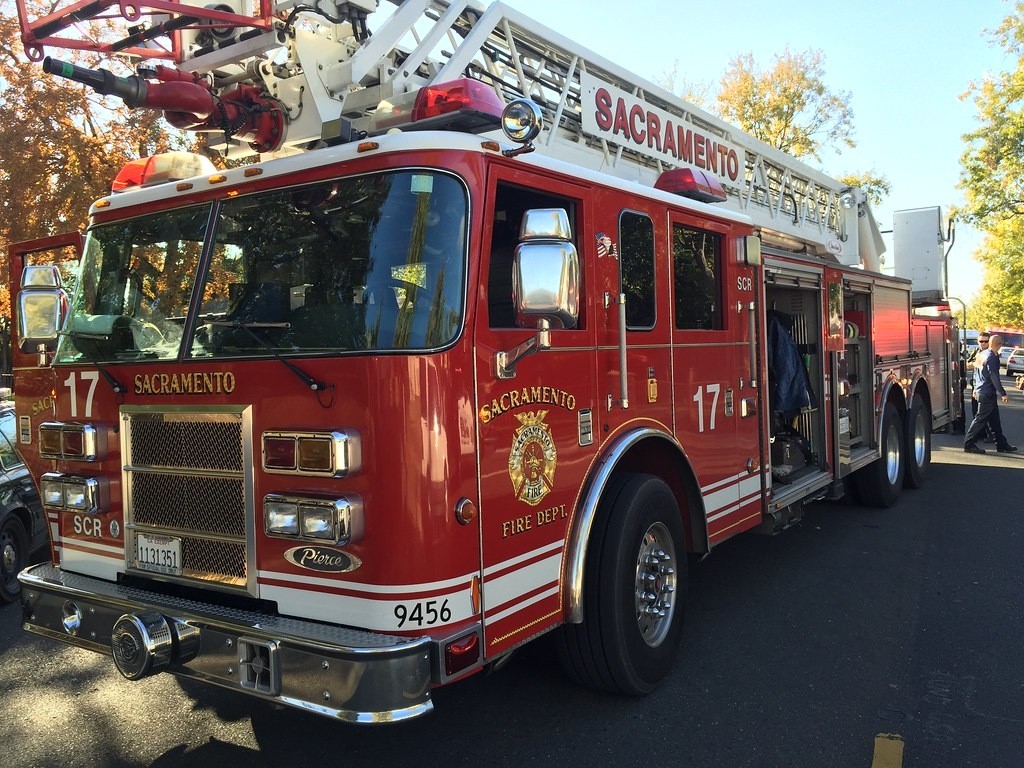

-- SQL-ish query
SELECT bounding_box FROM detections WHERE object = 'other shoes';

[951,431,967,436]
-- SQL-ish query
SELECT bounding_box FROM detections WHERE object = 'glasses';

[978,339,989,343]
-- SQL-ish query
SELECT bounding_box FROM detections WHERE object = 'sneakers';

[963,446,985,454]
[984,437,995,443]
[996,445,1017,452]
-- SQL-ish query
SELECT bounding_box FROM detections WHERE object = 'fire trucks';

[0,0,978,728]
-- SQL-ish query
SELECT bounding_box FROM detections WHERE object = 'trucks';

[959,329,980,357]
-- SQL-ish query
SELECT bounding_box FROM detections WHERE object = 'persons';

[950,332,1017,454]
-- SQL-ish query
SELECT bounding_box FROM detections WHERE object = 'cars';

[1006,348,1024,377]
[997,346,1015,367]
[1,397,51,607]
